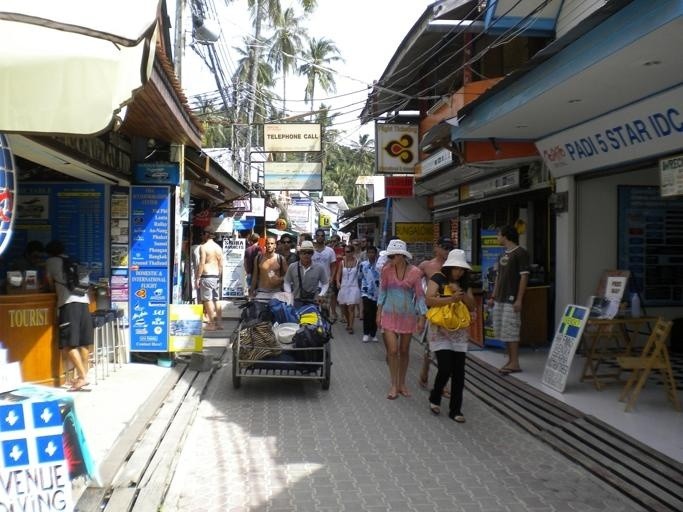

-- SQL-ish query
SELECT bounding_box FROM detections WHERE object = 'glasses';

[387,255,395,259]
[281,234,365,255]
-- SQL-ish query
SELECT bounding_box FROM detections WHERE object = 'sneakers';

[339,319,353,334]
[203,322,224,331]
[362,335,369,342]
[372,336,378,342]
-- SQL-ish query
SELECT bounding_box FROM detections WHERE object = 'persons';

[246,230,380,342]
[188,231,223,331]
[488,226,530,376]
[374,237,475,423]
[14,240,94,390]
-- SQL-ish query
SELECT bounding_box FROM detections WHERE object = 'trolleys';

[231,290,332,392]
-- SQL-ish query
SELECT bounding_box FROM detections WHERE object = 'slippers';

[67,382,91,392]
[498,365,522,373]
[387,376,451,400]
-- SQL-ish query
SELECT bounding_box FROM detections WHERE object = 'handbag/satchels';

[425,285,471,332]
[300,288,315,299]
[232,320,282,368]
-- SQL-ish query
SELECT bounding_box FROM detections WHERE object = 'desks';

[469,285,551,350]
[579,318,661,391]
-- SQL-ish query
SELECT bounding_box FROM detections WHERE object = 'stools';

[95,311,116,378]
[100,309,128,368]
[89,313,105,386]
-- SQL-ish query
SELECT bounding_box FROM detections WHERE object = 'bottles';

[632,293,640,317]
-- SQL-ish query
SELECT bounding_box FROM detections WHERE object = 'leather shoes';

[454,416,465,422]
[430,403,441,414]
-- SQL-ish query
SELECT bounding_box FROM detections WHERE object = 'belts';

[201,275,220,278]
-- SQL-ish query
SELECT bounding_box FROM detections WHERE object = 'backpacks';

[268,298,334,374]
[238,301,274,322]
[50,253,90,296]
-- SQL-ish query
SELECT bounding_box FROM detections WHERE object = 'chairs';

[616,316,681,413]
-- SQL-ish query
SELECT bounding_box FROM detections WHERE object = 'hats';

[199,226,215,235]
[379,239,413,260]
[442,248,472,271]
